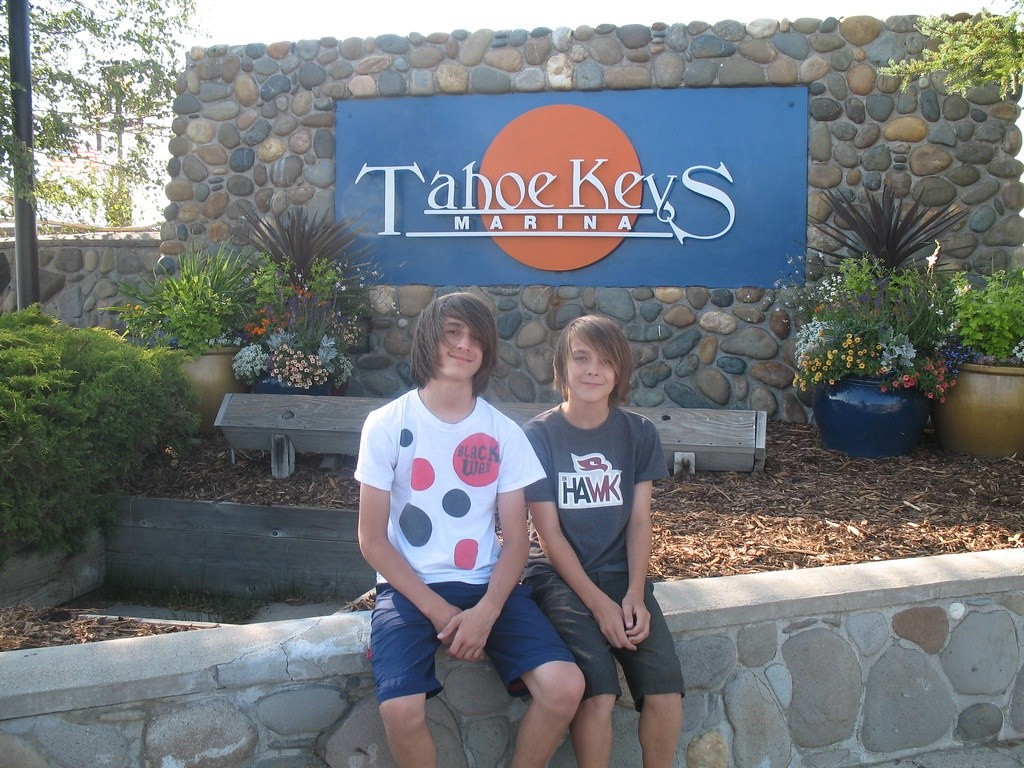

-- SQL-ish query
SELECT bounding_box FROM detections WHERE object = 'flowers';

[92,239,274,357]
[742,243,957,402]
[230,246,401,394]
[917,250,1024,369]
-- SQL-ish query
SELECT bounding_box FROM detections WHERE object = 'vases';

[926,362,1024,458]
[812,372,932,457]
[254,352,334,396]
[178,343,248,436]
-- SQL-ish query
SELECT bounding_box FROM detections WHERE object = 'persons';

[354,292,588,768]
[523,314,686,768]
